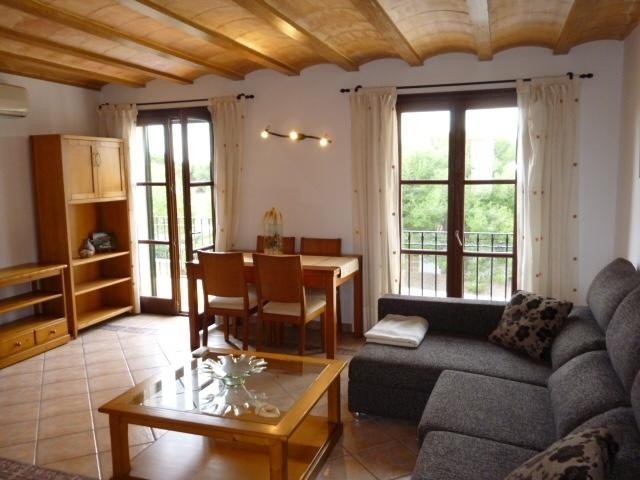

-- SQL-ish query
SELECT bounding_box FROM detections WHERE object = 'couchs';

[347,258,640,480]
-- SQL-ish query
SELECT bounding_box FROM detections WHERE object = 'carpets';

[0,456,102,480]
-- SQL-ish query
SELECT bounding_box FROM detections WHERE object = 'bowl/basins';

[196,352,270,388]
[193,383,269,416]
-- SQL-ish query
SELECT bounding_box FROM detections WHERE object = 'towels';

[363,314,429,348]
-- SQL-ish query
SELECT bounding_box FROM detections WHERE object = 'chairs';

[197,233,342,355]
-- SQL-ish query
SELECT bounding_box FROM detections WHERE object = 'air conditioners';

[0,81,29,119]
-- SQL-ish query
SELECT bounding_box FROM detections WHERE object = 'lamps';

[261,129,331,147]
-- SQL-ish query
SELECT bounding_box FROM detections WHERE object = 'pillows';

[504,419,616,480]
[487,289,573,365]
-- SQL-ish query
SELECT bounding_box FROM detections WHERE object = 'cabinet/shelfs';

[29,133,141,339]
[0,262,71,368]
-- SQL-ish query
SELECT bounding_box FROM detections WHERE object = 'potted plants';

[262,206,284,256]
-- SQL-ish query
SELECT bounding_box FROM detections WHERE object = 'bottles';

[78,238,95,259]
